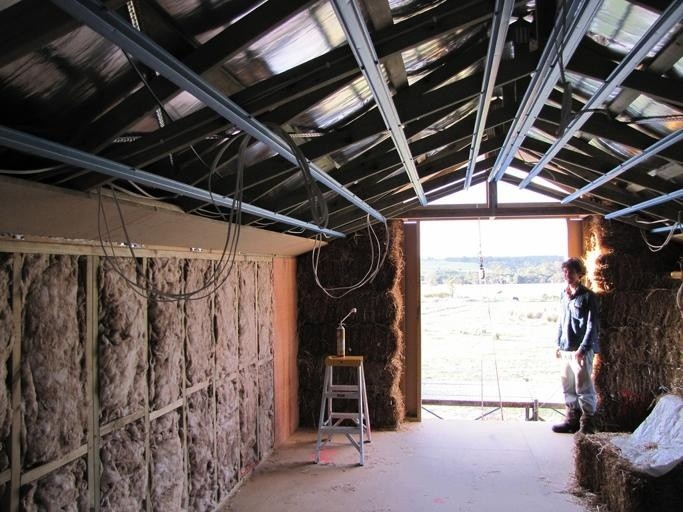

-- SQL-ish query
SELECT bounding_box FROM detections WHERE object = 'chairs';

[571,393,683,511]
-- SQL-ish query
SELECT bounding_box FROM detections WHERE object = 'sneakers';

[578,426,593,434]
[552,422,578,434]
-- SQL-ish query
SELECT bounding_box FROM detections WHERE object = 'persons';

[550,256,598,433]
